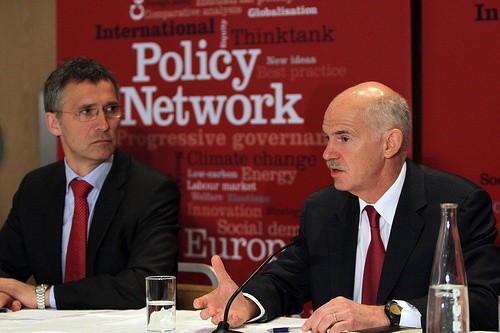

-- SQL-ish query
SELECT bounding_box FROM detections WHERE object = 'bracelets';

[36,285,48,309]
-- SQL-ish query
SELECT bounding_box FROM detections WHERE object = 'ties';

[362,205,385,305]
[64,179,94,283]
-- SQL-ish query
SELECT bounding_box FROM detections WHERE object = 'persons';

[193,81,500,333]
[0,58,180,310]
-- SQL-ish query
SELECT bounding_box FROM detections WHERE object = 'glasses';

[52,105,122,122]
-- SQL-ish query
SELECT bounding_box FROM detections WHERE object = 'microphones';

[212,236,307,333]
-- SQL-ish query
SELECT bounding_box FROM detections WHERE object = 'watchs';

[385,301,401,326]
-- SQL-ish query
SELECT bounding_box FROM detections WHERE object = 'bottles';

[426,203,469,333]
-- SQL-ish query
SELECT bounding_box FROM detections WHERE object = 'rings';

[333,313,337,320]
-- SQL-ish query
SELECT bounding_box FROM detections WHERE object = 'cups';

[145,276,177,332]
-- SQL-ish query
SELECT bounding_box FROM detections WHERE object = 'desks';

[0,308,425,333]
